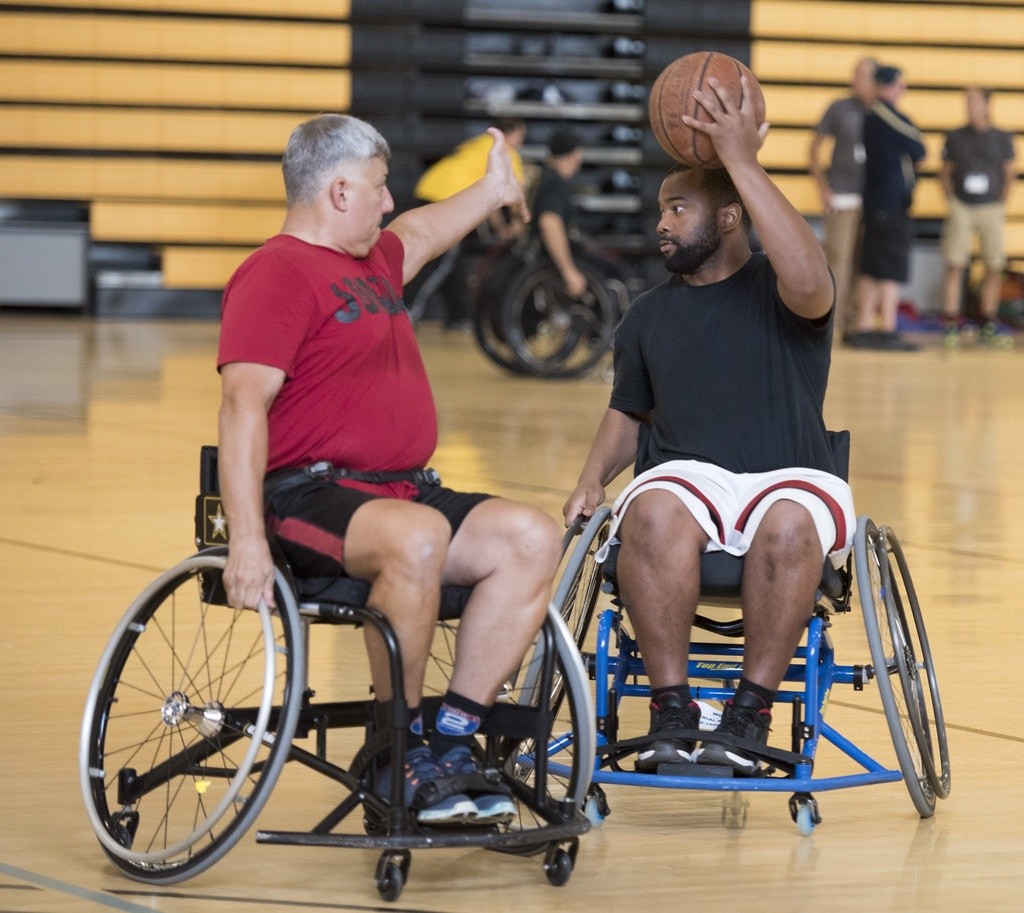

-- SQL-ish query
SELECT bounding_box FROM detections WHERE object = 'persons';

[402,117,653,382]
[940,87,1017,345]
[217,114,564,825]
[809,58,892,349]
[562,76,857,775]
[846,66,925,351]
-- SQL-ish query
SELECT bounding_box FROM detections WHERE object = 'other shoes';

[844,329,915,351]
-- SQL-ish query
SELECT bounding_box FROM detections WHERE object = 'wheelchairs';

[477,233,624,381]
[512,504,952,836]
[81,445,593,904]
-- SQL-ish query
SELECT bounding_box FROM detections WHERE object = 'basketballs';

[650,50,764,168]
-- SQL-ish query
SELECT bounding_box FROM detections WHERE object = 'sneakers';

[633,691,701,772]
[369,742,518,826]
[696,690,773,775]
